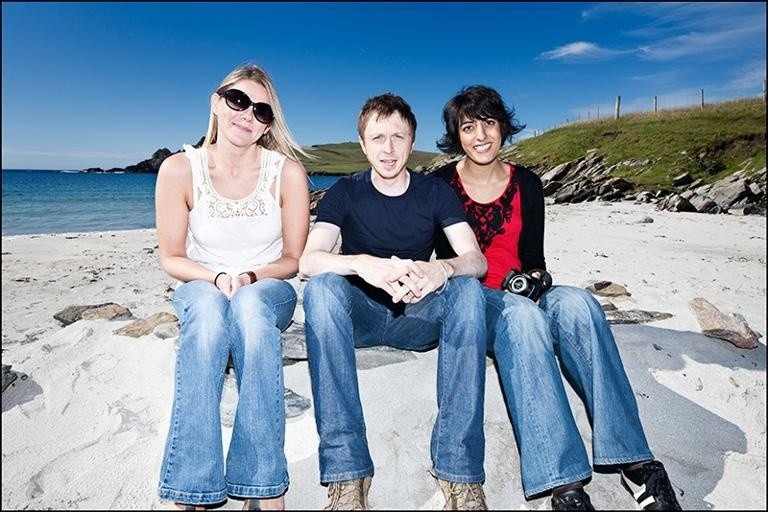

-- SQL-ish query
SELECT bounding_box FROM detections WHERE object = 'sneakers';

[326,469,372,511]
[621,461,682,510]
[551,486,596,511]
[434,471,490,511]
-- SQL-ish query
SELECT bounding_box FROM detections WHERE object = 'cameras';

[501,267,544,303]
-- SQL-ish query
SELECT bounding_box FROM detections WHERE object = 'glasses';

[219,89,275,124]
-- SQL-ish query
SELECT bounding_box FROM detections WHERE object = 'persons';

[297,92,498,510]
[154,62,313,510]
[431,86,686,511]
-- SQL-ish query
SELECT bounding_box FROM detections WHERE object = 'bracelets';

[238,271,259,283]
[212,271,228,285]
[437,259,449,295]
